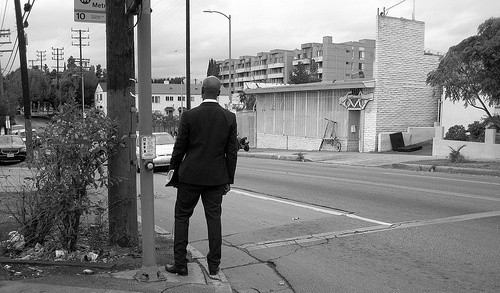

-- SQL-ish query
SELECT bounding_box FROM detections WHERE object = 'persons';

[165,76,238,276]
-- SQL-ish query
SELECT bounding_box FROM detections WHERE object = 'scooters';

[236,133,250,152]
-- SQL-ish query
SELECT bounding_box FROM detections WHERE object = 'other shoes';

[210,269,219,275]
[165,263,188,276]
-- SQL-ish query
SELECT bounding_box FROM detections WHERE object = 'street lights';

[203,10,231,103]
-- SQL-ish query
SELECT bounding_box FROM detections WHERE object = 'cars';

[15,128,42,148]
[9,125,25,135]
[135,132,186,173]
[0,135,27,163]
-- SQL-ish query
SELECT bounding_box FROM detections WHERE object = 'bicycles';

[319,117,342,153]
